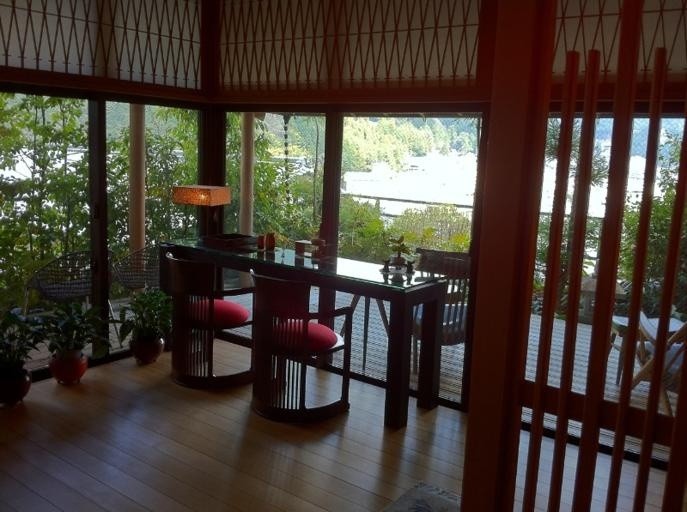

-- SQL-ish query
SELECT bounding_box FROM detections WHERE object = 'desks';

[156,233,449,433]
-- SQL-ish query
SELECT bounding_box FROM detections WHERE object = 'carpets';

[381,481,464,512]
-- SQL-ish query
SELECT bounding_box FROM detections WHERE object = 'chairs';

[163,250,354,426]
[23,243,163,349]
[404,244,471,374]
[622,306,687,418]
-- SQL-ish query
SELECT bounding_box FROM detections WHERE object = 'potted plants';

[1,289,175,406]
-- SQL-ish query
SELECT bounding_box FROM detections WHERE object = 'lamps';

[173,184,234,247]
